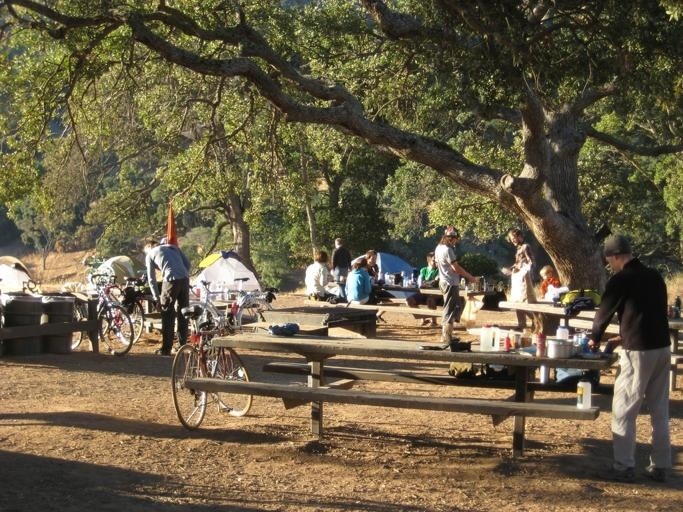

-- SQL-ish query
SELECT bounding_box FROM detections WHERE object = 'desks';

[497,298,683,390]
[334,282,497,298]
[82,290,199,333]
[208,331,617,460]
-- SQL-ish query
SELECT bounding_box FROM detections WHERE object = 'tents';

[0,254,37,295]
[187,249,262,302]
[348,252,418,300]
[90,254,147,285]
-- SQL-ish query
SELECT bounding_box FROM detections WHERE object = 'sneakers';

[596,465,638,483]
[150,344,180,357]
[639,463,668,482]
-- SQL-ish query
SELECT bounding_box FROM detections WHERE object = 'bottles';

[539,365,549,385]
[477,323,613,359]
[576,377,591,412]
[673,296,681,319]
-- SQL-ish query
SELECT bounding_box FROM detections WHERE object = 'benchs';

[183,374,600,426]
[302,298,442,319]
[532,333,681,368]
[263,360,578,394]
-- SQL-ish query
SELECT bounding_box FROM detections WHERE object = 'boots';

[440,323,460,343]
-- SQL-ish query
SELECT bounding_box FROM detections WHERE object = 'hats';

[442,226,460,238]
[600,235,633,258]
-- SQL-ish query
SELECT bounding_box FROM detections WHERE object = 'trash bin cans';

[3,294,76,355]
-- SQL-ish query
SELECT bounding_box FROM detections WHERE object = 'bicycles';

[71,272,278,431]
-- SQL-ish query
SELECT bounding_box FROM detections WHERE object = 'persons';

[405,252,443,326]
[330,237,352,283]
[303,250,346,304]
[140,238,194,359]
[538,265,560,335]
[586,234,673,484]
[499,229,540,335]
[433,225,481,348]
[343,257,382,306]
[363,250,395,298]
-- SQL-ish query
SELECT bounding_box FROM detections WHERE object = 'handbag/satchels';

[554,366,602,388]
[559,286,601,317]
[484,364,537,381]
[269,322,301,337]
[447,343,476,379]
[118,287,136,315]
